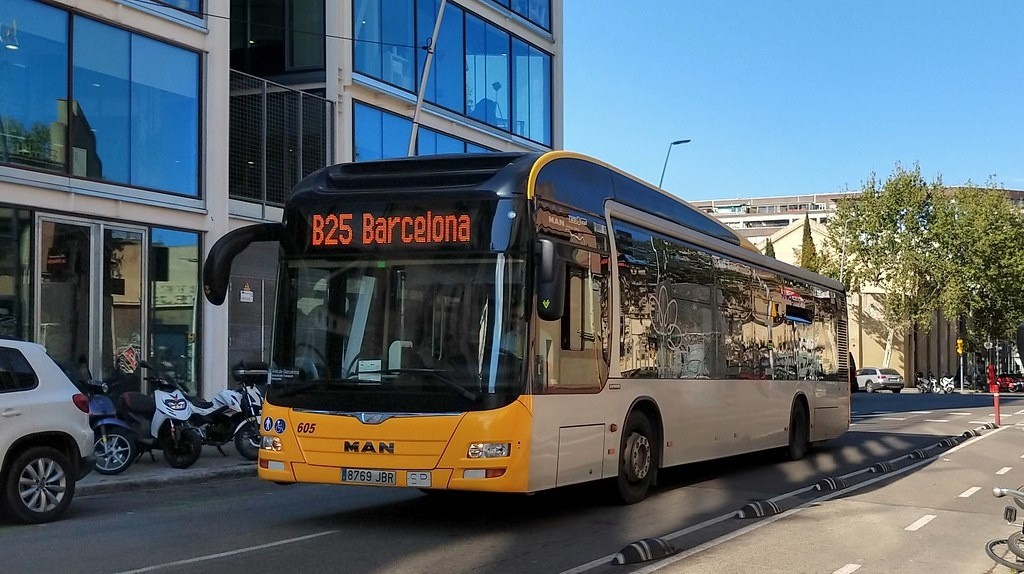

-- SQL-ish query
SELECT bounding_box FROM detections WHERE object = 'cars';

[855,367,904,394]
[0,337,99,526]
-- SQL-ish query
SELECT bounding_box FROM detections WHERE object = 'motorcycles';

[914,370,943,394]
[108,359,206,469]
[175,359,269,462]
[938,371,955,395]
[61,353,138,476]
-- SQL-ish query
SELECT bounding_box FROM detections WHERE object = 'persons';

[500,302,526,358]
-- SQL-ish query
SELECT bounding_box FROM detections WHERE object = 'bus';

[197,147,854,507]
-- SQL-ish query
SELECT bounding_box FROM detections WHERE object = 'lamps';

[5,20,20,50]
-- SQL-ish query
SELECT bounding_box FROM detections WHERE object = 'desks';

[384,51,412,84]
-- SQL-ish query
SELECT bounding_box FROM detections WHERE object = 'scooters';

[994,372,1023,392]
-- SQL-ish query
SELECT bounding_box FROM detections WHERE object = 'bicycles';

[984,483,1024,572]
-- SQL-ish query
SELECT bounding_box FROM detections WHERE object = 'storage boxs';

[232,361,268,384]
